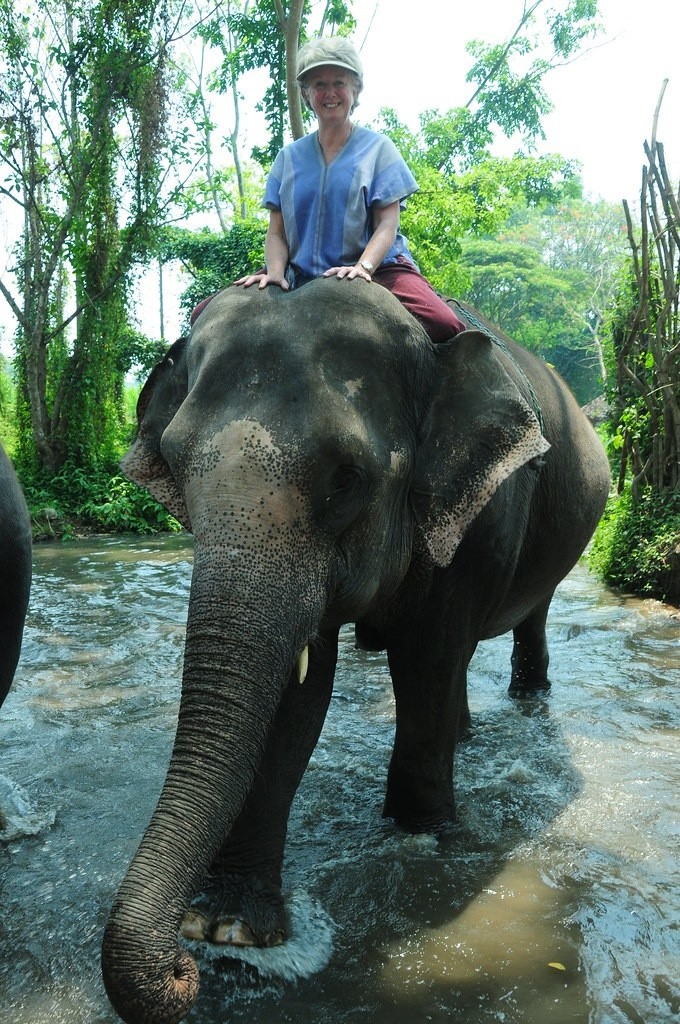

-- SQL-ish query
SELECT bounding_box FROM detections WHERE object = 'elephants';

[100,276,610,1024]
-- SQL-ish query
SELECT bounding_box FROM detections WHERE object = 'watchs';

[359,260,374,273]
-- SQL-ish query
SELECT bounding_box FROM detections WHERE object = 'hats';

[296,59,359,80]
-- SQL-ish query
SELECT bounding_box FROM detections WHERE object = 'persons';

[189,35,465,346]
